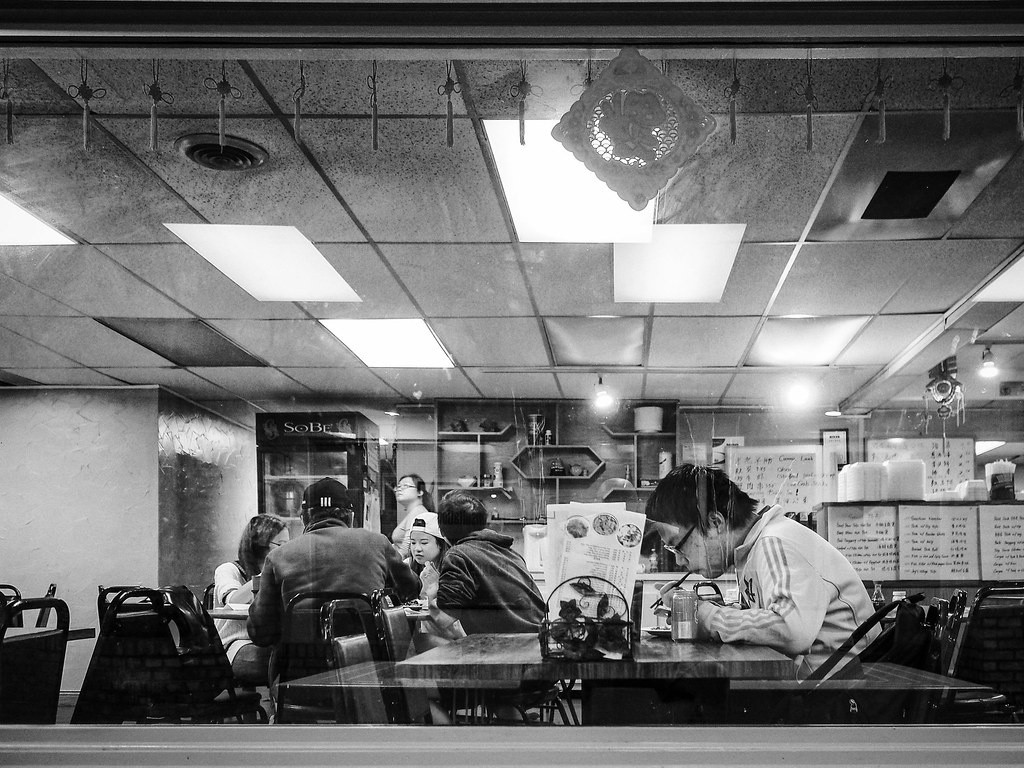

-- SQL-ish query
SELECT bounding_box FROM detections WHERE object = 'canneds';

[670,588,698,642]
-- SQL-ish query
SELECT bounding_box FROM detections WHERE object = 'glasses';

[393,484,418,492]
[663,513,709,555]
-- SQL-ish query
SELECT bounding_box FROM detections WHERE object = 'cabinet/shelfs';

[393,397,682,555]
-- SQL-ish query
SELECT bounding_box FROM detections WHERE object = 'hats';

[411,511,454,546]
[301,476,355,511]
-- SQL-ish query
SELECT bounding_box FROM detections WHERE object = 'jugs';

[568,463,582,476]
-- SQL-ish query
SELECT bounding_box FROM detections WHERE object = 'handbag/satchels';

[890,599,942,676]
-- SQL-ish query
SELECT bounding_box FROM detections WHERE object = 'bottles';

[659,451,673,480]
[481,474,492,487]
[892,591,907,611]
[545,429,552,445]
[491,507,500,520]
[649,548,658,573]
[492,462,502,480]
[871,584,885,612]
[526,413,544,445]
[550,458,567,477]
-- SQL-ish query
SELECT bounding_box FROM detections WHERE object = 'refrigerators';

[255,412,382,533]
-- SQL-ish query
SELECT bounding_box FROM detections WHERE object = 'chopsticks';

[650,571,693,610]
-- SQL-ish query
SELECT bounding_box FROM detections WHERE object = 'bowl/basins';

[837,458,987,501]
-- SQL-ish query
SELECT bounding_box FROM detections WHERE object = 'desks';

[206,608,437,621]
[394,630,797,726]
[4,626,96,642]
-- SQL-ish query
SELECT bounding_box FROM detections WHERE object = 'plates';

[564,512,642,548]
[641,626,671,636]
[404,606,422,611]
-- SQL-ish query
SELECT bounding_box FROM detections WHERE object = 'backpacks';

[139,583,233,701]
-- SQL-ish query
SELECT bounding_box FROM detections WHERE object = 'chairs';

[0,580,1024,726]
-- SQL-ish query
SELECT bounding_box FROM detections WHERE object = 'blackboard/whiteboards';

[864,435,975,492]
[724,445,822,514]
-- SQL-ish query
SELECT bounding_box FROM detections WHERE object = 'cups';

[637,563,646,573]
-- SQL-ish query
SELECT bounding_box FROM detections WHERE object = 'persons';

[246,477,422,704]
[391,473,435,561]
[402,512,467,653]
[404,489,545,725]
[646,464,882,680]
[214,514,290,685]
